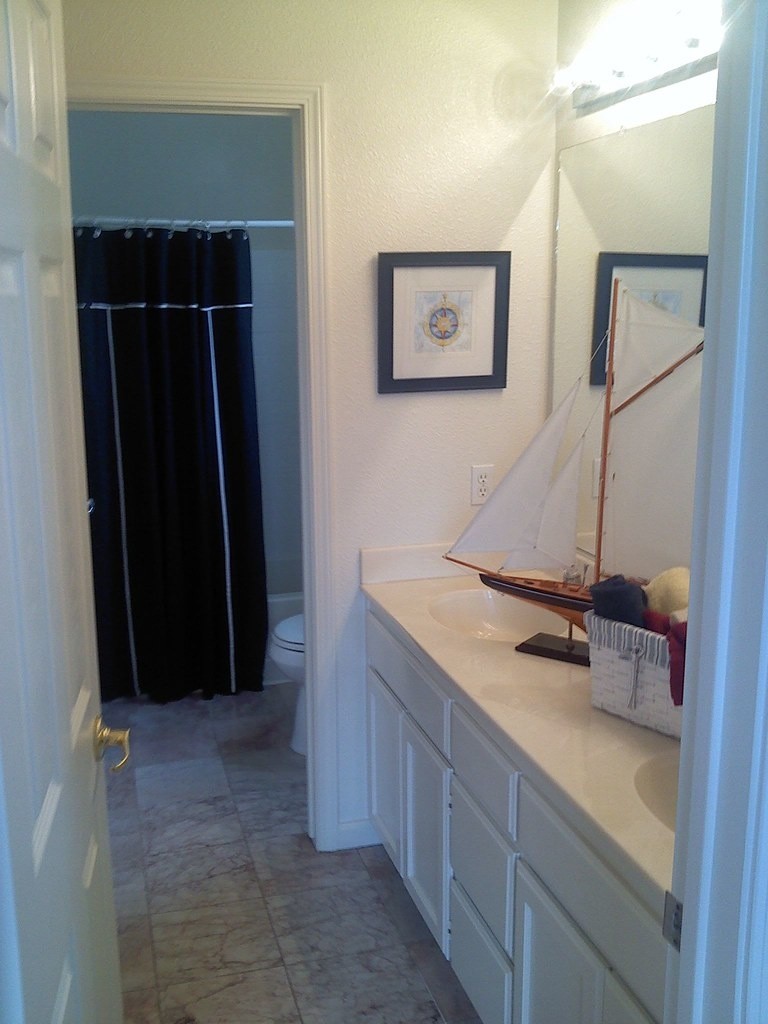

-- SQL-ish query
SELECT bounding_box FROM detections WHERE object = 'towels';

[590,565,691,706]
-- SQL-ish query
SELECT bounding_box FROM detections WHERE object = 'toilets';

[268,614,309,758]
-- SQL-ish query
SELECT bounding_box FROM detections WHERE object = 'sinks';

[634,749,681,835]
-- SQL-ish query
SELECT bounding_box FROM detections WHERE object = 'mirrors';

[545,48,711,587]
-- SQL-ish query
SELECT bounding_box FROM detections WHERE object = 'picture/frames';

[589,252,709,385]
[376,250,512,394]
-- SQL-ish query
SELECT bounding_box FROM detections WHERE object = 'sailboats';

[442,277,706,641]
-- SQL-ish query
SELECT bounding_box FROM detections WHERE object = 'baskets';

[584,609,681,739]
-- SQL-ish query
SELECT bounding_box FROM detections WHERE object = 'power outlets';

[591,458,614,498]
[471,465,493,505]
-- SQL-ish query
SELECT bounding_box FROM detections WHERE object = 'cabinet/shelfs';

[366,618,673,1024]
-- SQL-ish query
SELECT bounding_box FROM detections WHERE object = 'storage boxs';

[583,583,689,740]
